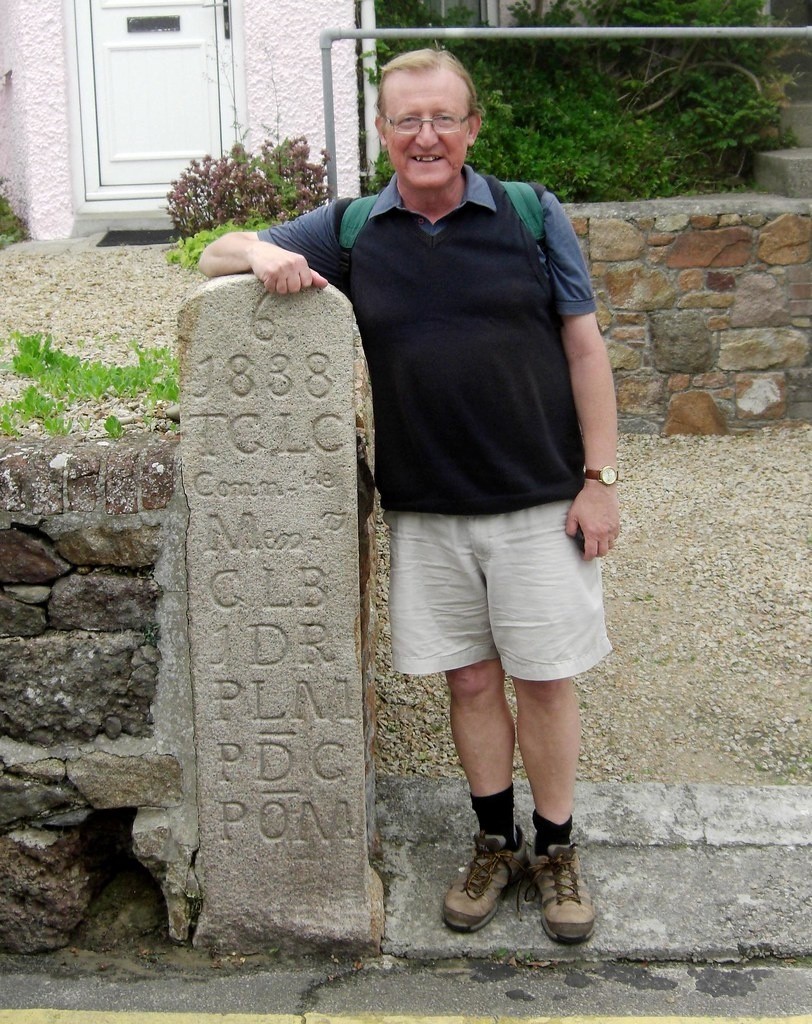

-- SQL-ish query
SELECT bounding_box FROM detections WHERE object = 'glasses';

[381,114,471,134]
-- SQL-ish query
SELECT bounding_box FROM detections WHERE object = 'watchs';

[584,465,619,486]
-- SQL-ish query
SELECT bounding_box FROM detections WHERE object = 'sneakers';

[517,831,596,946]
[441,824,532,934]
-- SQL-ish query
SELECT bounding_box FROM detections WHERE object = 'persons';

[190,48,621,946]
